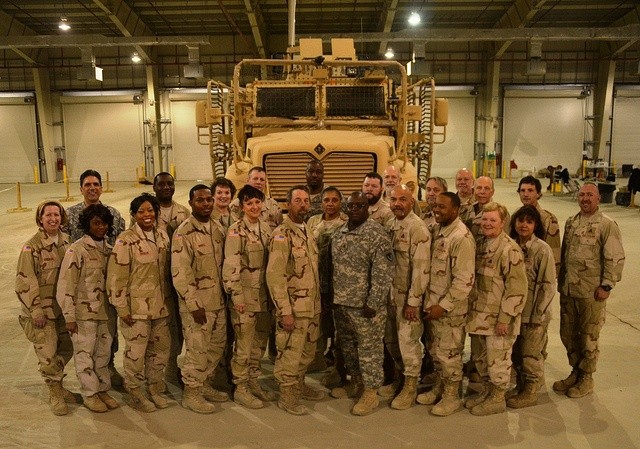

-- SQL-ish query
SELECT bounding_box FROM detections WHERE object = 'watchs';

[601,285,612,292]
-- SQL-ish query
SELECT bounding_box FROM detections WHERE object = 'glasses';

[346,201,368,210]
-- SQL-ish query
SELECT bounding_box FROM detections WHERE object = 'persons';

[557,182,625,398]
[510,176,560,277]
[128,172,190,228]
[221,185,275,409]
[305,186,349,234]
[55,204,118,413]
[229,167,284,226]
[463,202,527,416]
[210,177,237,225]
[171,184,229,414]
[421,176,448,217]
[462,176,510,233]
[417,192,477,416]
[504,206,556,409]
[15,200,81,416]
[455,169,475,204]
[377,184,431,410]
[64,169,125,237]
[318,192,395,416]
[380,165,423,217]
[361,172,393,224]
[106,192,169,412]
[266,185,325,415]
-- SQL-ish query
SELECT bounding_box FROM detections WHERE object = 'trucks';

[195,38,447,210]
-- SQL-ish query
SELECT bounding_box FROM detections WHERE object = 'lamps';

[59,15,71,33]
[408,11,420,27]
[385,45,395,59]
[131,50,140,64]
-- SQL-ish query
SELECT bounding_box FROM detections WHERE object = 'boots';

[130,387,156,413]
[302,384,325,400]
[233,385,263,409]
[352,390,379,415]
[62,387,83,404]
[81,394,107,412]
[149,391,169,409]
[391,376,417,410]
[471,386,506,416]
[48,380,68,416]
[331,383,358,398]
[99,391,118,409]
[251,381,275,401]
[430,382,461,417]
[505,377,525,401]
[202,381,229,402]
[553,369,579,395]
[567,373,593,398]
[465,384,490,409]
[181,389,216,414]
[379,380,401,396]
[278,384,306,415]
[417,382,442,405]
[506,379,538,409]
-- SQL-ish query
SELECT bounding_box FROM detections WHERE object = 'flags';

[276,235,285,240]
[66,248,74,254]
[229,230,238,236]
[176,233,183,239]
[23,245,32,252]
[116,240,122,246]
